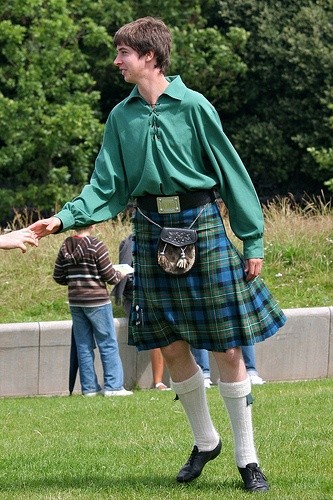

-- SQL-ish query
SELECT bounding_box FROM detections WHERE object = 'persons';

[26,15,287,490]
[52,223,265,397]
[0,229,39,253]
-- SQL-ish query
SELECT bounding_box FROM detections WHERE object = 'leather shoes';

[237,463,269,492]
[176,437,221,482]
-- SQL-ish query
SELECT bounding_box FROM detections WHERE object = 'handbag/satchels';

[158,227,197,274]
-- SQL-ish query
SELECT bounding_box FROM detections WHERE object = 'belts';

[133,188,216,214]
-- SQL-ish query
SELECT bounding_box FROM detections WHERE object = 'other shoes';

[250,376,266,385]
[152,382,171,391]
[103,388,133,397]
[84,389,102,397]
[202,378,212,389]
